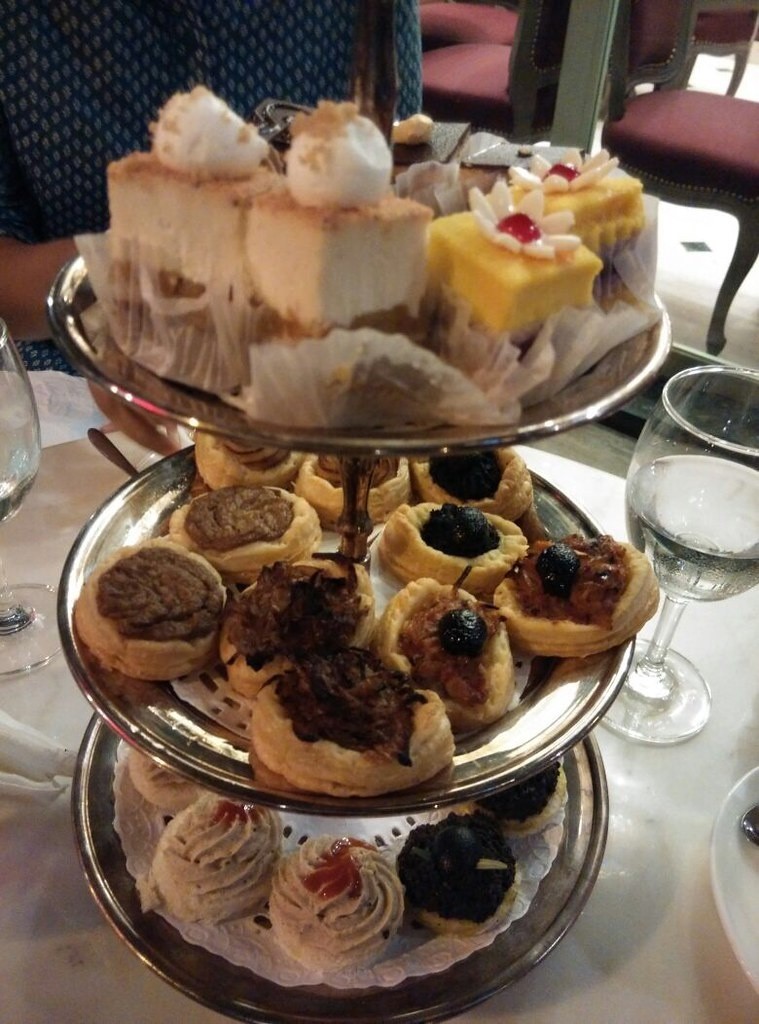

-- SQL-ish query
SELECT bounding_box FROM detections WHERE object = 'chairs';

[420,0,759,355]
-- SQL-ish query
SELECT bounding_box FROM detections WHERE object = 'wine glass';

[599,365,759,743]
[0,317,63,681]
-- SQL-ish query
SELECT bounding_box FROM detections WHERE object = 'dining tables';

[0,431,759,1024]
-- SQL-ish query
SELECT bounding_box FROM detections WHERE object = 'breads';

[73,425,660,797]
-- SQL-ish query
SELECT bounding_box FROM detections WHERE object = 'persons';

[0,1,422,377]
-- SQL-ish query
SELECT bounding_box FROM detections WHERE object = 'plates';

[708,764,759,997]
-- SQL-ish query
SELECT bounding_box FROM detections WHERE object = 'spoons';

[740,804,759,849]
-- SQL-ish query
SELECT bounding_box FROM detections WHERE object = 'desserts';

[126,747,566,972]
[72,85,660,423]
